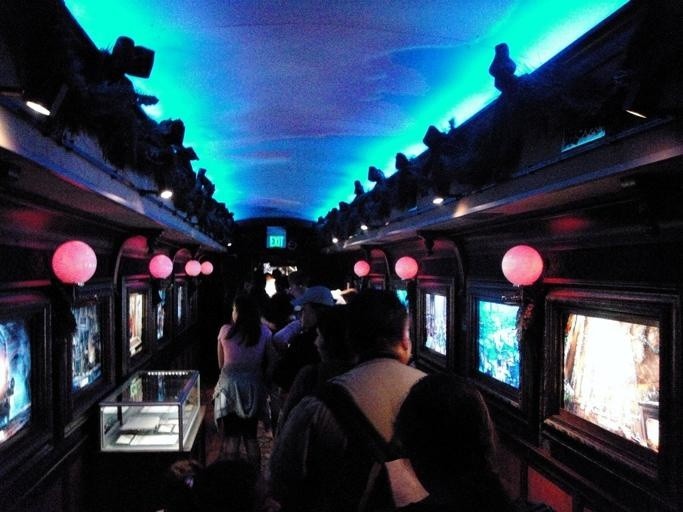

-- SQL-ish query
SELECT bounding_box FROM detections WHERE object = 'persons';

[211,267,357,470]
[266,289,527,512]
[171,456,282,512]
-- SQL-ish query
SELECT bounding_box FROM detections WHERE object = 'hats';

[290,286,335,306]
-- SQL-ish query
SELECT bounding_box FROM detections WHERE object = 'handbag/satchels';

[263,347,281,386]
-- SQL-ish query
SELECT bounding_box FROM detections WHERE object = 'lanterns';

[355,261,369,277]
[502,245,543,286]
[395,256,417,280]
